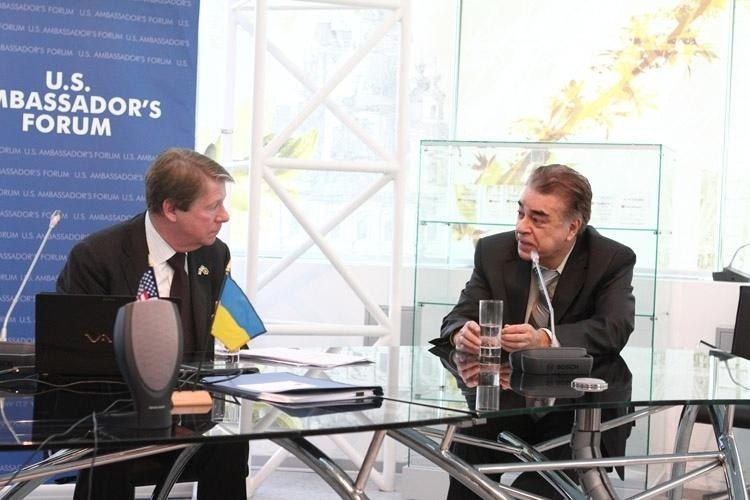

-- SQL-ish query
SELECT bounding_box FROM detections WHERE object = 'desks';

[1,345,750,499]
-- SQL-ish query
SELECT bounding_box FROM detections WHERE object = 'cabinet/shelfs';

[408,141,680,491]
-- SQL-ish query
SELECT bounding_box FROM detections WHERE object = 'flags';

[209,272,268,353]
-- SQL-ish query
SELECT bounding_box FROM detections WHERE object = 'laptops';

[36,291,182,378]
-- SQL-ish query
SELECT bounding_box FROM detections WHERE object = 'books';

[238,347,378,370]
[263,397,384,418]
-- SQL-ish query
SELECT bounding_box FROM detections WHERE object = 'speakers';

[113,301,184,429]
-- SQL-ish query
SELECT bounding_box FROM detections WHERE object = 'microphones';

[508,247,595,374]
[0,209,65,375]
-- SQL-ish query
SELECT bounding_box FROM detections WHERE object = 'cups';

[475,371,502,411]
[479,299,504,366]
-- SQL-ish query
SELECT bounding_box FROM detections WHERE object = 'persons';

[438,344,635,415]
[53,148,252,498]
[426,162,639,499]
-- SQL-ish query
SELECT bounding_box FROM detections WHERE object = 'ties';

[527,267,553,330]
[161,251,199,359]
